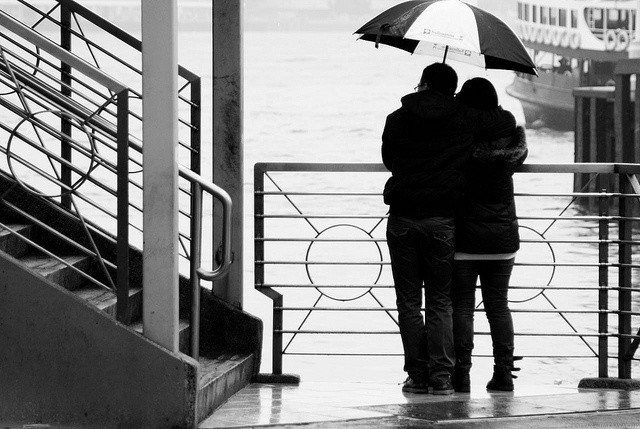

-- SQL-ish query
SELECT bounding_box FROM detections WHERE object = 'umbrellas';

[354,1,538,78]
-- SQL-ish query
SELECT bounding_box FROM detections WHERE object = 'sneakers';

[432,381,454,396]
[487,365,519,392]
[398,376,429,395]
[451,370,470,393]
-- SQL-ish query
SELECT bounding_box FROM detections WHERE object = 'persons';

[451,78,528,392]
[382,63,515,395]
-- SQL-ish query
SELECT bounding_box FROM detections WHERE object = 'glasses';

[414,84,426,93]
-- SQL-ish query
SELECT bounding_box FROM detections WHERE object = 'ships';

[506,1,640,131]
